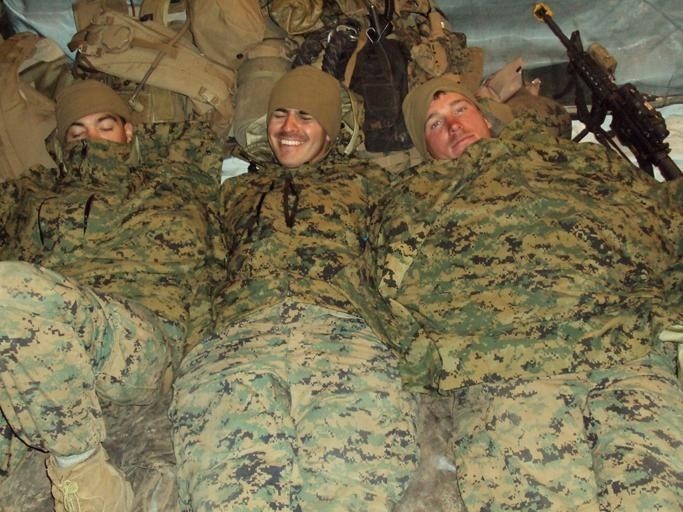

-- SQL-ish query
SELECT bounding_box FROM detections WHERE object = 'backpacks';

[63,0,303,147]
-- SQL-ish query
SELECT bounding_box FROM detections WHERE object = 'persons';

[0,78,227,511]
[329,77,682,512]
[170,64,417,511]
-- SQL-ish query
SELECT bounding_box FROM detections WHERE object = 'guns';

[534,3,683,181]
[366,0,394,40]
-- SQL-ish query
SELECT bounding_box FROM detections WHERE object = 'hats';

[399,77,484,161]
[264,62,342,144]
[51,79,131,147]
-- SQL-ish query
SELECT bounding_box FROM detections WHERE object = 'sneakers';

[43,442,135,512]
